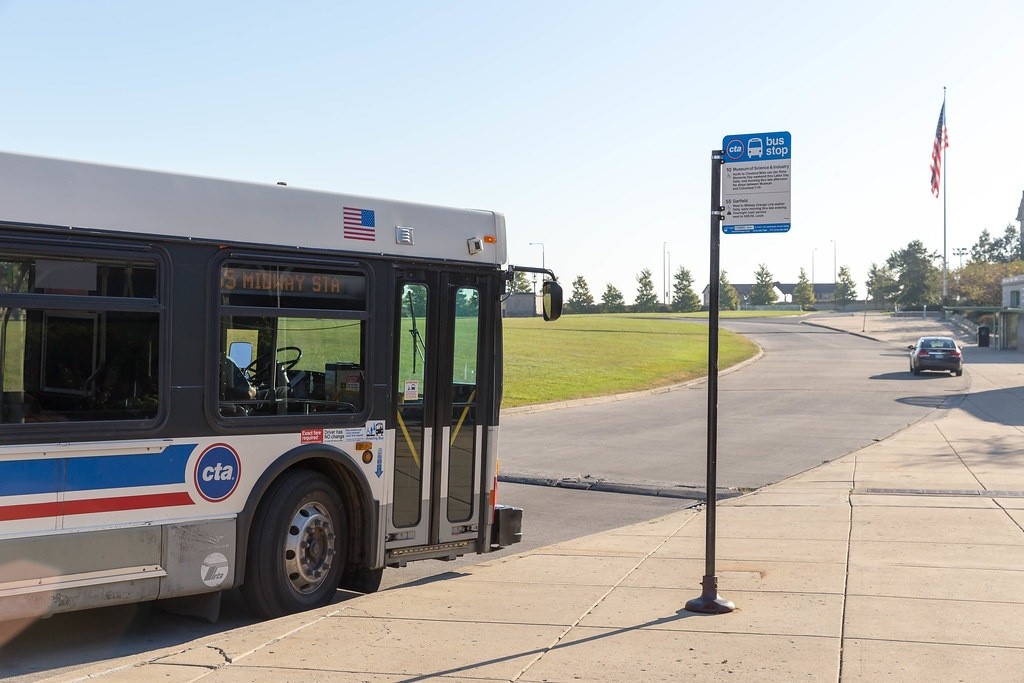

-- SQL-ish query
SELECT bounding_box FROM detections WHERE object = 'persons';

[219,348,294,415]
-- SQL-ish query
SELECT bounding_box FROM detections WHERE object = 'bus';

[0,145,566,632]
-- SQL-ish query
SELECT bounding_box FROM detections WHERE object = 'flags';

[928,103,950,199]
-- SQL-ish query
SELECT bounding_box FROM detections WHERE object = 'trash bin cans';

[977,326,989,348]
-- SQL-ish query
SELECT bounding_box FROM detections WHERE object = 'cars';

[908,336,963,377]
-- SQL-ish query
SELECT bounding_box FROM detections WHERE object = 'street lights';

[830,239,837,309]
[812,247,818,294]
[528,242,544,284]
[667,252,671,305]
[663,241,665,305]
[953,247,968,301]
